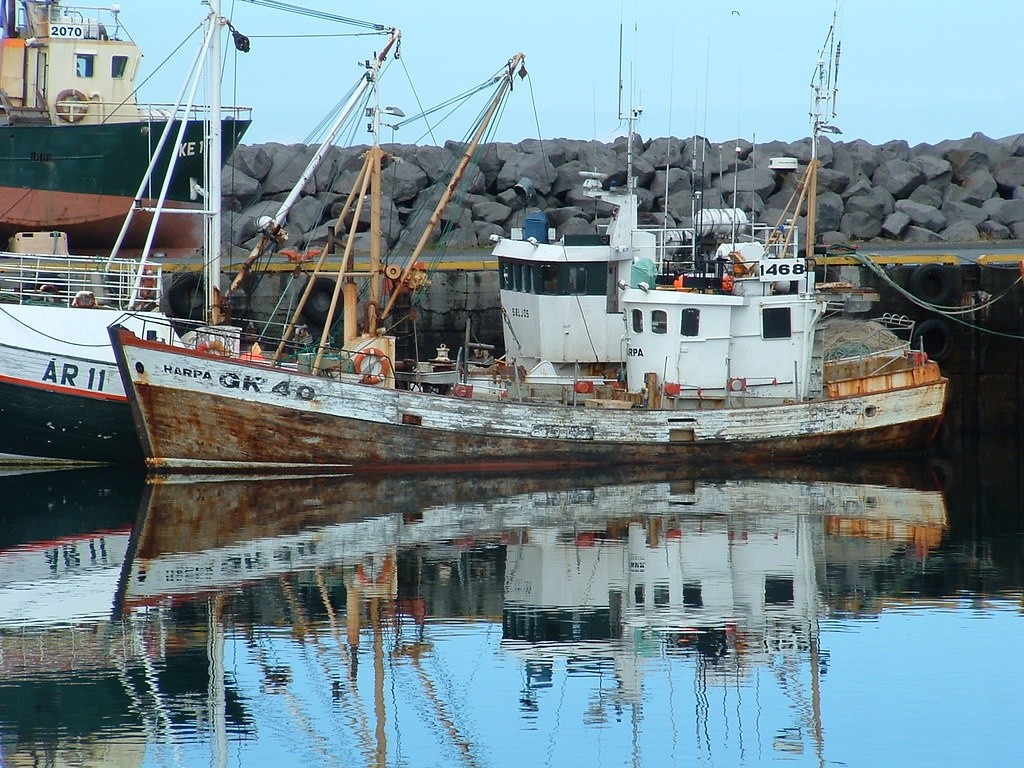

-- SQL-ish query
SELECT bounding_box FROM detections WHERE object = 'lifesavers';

[196,341,229,357]
[354,348,390,385]
[74,291,96,309]
[140,264,154,299]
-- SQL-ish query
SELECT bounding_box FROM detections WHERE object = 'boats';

[0,461,185,768]
[102,452,951,768]
[1,1,253,251]
[105,1,955,484]
[1,2,460,472]
